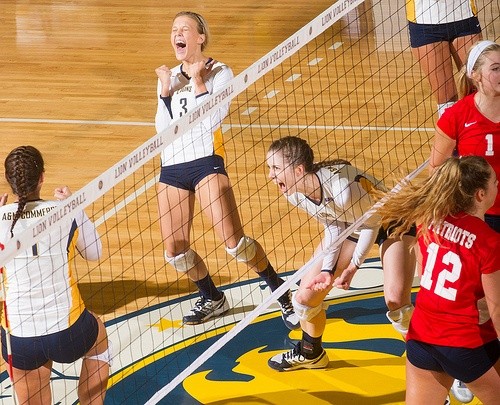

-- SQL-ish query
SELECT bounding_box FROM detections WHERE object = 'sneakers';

[268,340,329,372]
[183,291,230,325]
[277,287,301,330]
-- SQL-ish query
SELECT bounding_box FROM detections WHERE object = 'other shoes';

[444,393,451,405]
[452,378,473,403]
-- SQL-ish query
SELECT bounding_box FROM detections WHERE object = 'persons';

[0,145,113,405]
[155,10,300,331]
[373,150,500,405]
[267,136,450,405]
[430,41,500,402]
[406,0,484,119]
